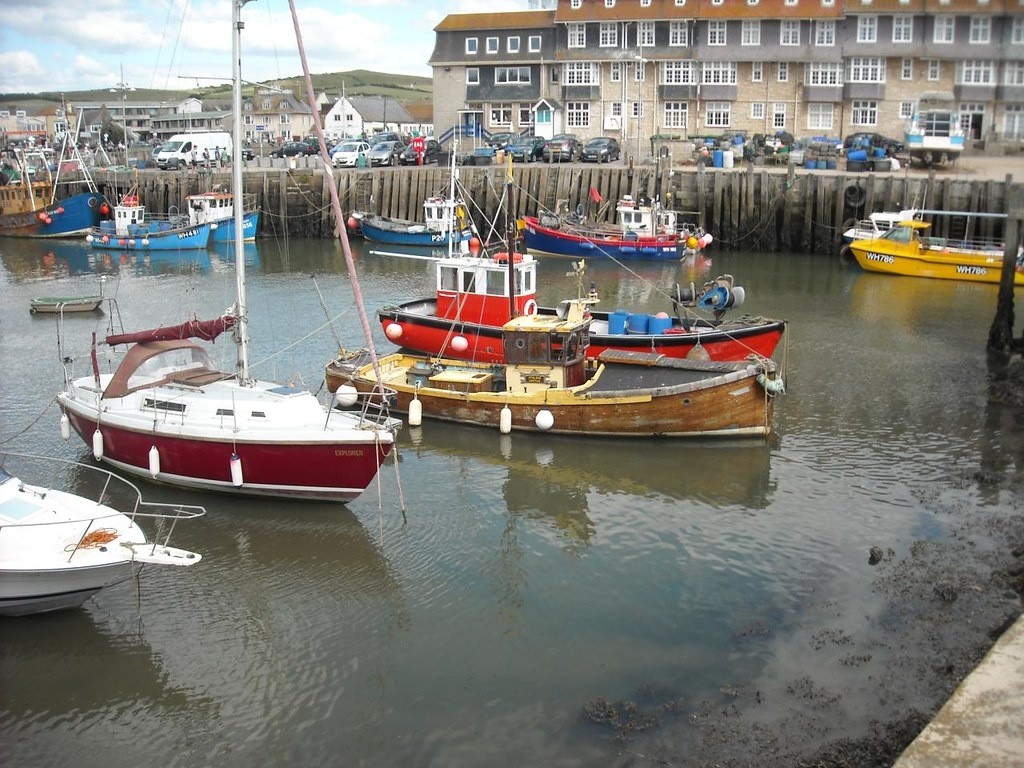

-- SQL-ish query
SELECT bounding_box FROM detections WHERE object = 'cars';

[269,136,327,156]
[326,131,441,168]
[12,147,58,174]
[486,132,621,164]
[137,141,150,147]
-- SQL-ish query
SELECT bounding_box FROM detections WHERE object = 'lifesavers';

[493,253,524,264]
[844,184,866,207]
[839,244,852,257]
[842,217,860,234]
[524,299,538,317]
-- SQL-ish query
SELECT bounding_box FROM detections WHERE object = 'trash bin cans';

[358,153,365,168]
[128,158,137,166]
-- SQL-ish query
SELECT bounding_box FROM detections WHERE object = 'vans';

[156,132,234,170]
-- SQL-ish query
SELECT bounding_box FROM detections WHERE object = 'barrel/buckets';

[613,311,629,316]
[286,153,325,170]
[648,316,673,335]
[627,313,650,335]
[805,137,888,169]
[99,220,116,234]
[608,311,629,334]
[127,220,169,236]
[706,135,743,168]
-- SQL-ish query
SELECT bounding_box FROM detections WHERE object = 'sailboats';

[55,1,400,505]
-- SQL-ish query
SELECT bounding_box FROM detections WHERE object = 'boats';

[840,164,1024,287]
[522,128,713,263]
[27,282,103,313]
[320,150,792,437]
[86,168,263,251]
[0,90,107,238]
[1,450,209,621]
[346,123,476,245]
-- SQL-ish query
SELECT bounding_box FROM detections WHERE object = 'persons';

[244,142,252,149]
[329,139,338,149]
[203,148,211,168]
[307,145,314,157]
[222,147,230,170]
[191,145,197,174]
[215,146,221,173]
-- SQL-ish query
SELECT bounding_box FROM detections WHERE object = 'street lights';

[109,82,136,169]
